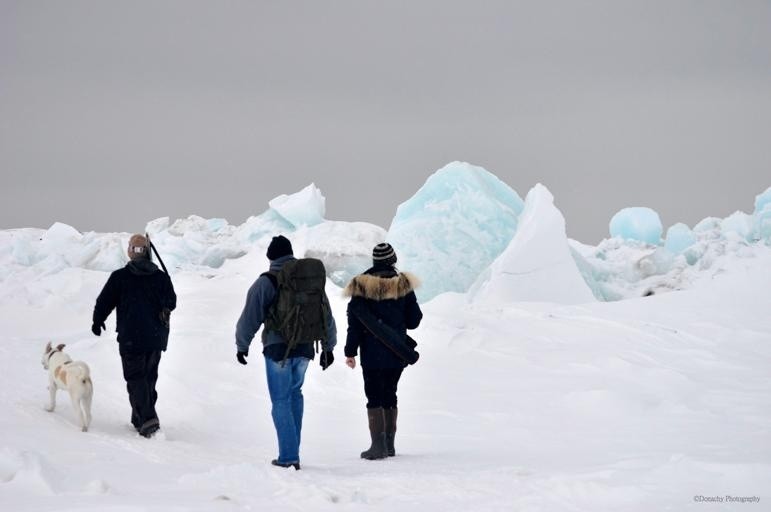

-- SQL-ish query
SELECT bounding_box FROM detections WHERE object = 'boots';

[361,408,397,460]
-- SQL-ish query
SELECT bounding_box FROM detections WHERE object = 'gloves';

[320,351,334,370]
[237,351,247,364]
[92,323,105,335]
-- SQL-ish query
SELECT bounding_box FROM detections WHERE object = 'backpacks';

[269,258,328,368]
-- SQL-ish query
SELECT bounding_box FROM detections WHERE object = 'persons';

[91,235,177,439]
[340,242,424,461]
[234,235,337,470]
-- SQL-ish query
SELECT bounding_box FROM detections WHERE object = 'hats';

[128,235,149,259]
[267,235,293,260]
[373,243,397,265]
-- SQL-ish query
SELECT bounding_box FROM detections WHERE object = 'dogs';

[41,341,94,432]
